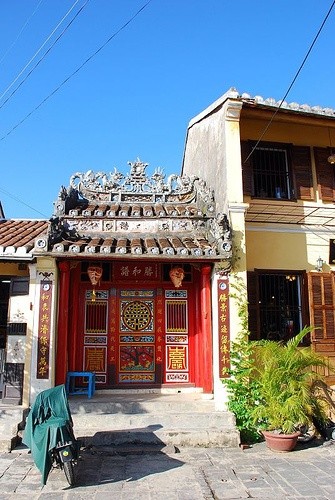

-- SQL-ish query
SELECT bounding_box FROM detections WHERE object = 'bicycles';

[22,384,80,487]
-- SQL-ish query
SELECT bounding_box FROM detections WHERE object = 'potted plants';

[236,326,335,450]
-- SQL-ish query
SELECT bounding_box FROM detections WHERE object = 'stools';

[66,371,95,400]
